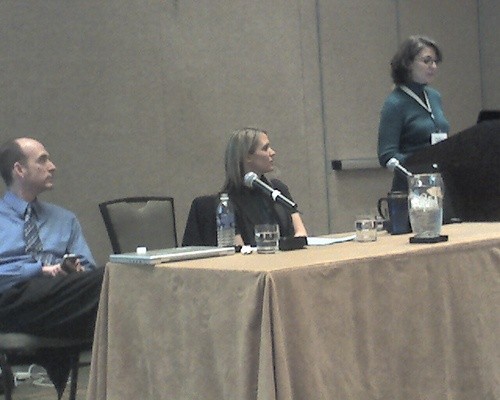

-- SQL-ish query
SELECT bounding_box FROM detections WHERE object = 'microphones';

[243,172,298,213]
[385,158,413,180]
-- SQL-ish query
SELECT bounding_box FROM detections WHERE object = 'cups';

[407,173,443,238]
[378,192,412,235]
[355,216,377,242]
[255,224,279,254]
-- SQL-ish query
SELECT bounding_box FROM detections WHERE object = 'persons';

[0,137,105,347]
[215,126,308,247]
[378,37,451,193]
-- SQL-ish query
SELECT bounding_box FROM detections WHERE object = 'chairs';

[98,196,179,254]
[0,328,95,400]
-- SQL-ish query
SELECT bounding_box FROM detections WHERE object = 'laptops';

[109,245,235,265]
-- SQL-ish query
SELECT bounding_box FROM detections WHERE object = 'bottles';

[216,193,236,248]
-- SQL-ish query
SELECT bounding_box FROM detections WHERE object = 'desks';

[85,221,500,400]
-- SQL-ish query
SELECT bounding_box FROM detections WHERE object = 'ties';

[23,203,44,266]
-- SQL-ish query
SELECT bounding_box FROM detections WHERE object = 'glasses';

[412,55,440,65]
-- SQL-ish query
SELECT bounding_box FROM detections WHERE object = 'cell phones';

[61,253,77,274]
